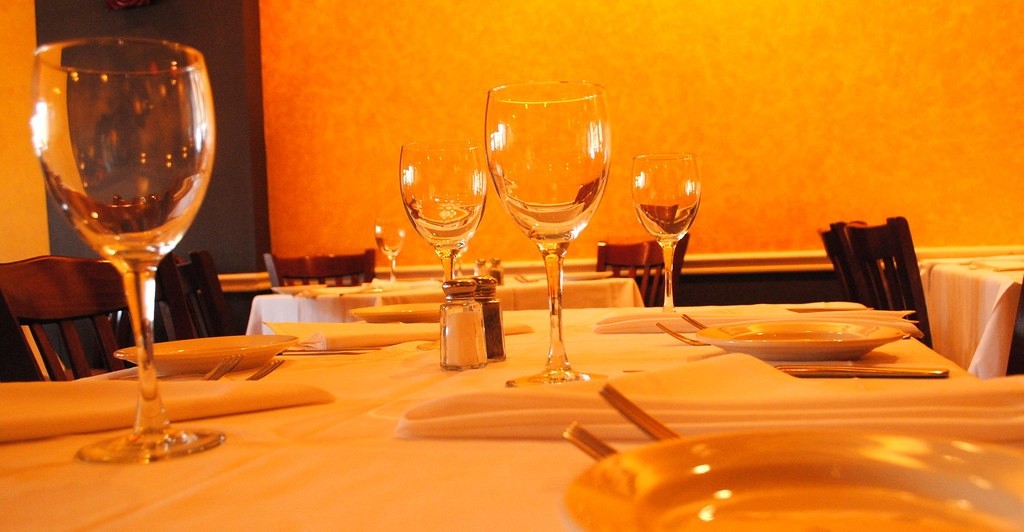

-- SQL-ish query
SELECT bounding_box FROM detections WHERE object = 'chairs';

[114,251,233,341]
[841,216,933,348]
[263,248,377,294]
[596,233,690,307]
[818,220,889,308]
[0,251,199,382]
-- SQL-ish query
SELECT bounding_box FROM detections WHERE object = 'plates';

[271,283,327,295]
[348,302,442,322]
[694,320,905,361]
[563,428,1024,532]
[113,334,298,375]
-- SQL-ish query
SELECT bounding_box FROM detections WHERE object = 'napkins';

[0,380,336,444]
[392,354,1024,446]
[261,318,535,350]
[592,307,924,340]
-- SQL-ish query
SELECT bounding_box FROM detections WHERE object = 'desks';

[244,277,644,336]
[917,255,1024,379]
[0,301,978,532]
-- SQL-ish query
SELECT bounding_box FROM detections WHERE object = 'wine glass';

[632,153,702,314]
[29,37,227,464]
[484,79,613,388]
[399,136,489,281]
[372,219,406,283]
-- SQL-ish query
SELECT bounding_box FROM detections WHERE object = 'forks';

[110,358,284,381]
[681,313,708,331]
[197,353,246,380]
[596,384,680,441]
[655,322,950,378]
[564,421,618,458]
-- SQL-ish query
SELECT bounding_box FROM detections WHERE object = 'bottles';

[489,257,503,285]
[439,278,488,370]
[473,258,487,277]
[471,275,506,363]
